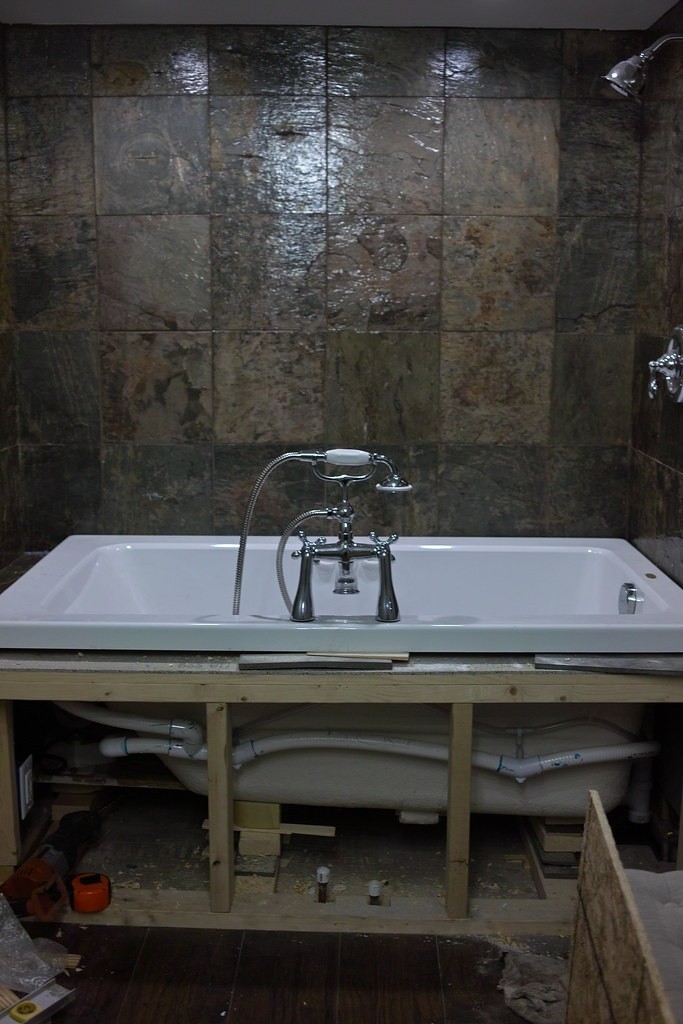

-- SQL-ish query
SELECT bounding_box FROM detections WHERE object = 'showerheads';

[600,51,650,109]
[376,473,414,494]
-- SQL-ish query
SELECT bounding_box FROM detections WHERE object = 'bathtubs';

[0,532,683,821]
